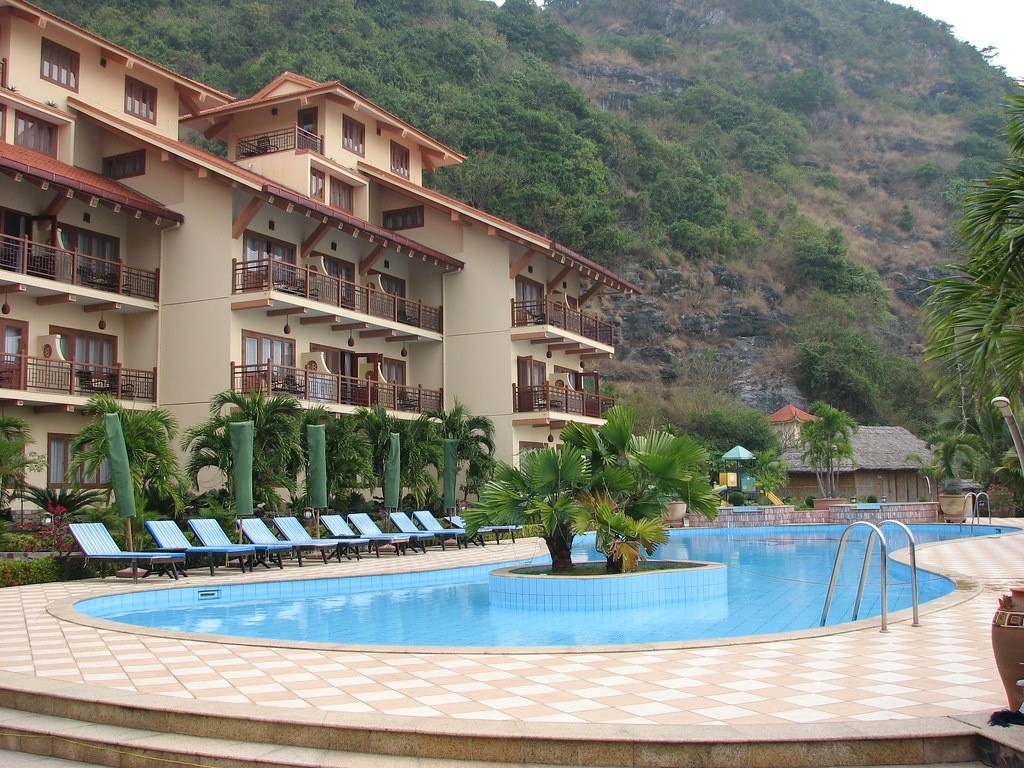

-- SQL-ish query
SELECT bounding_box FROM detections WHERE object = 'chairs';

[262,371,307,398]
[525,307,560,328]
[242,136,279,157]
[398,392,420,412]
[532,391,562,412]
[271,275,319,302]
[69,509,524,584]
[397,308,419,327]
[78,266,131,297]
[77,369,135,400]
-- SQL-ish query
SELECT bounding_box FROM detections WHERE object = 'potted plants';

[793,399,860,510]
[939,473,972,523]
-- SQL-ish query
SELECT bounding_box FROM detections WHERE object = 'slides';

[760,488,784,506]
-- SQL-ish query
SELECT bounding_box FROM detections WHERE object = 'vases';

[660,501,686,528]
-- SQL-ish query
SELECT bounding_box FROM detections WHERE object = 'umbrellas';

[444,439,460,530]
[229,420,253,544]
[105,413,136,552]
[384,433,400,533]
[308,424,327,539]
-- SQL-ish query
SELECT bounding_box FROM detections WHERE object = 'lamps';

[401,341,407,357]
[546,342,552,358]
[348,329,354,347]
[580,349,586,368]
[2,294,9,314]
[99,311,107,329]
[283,314,291,334]
